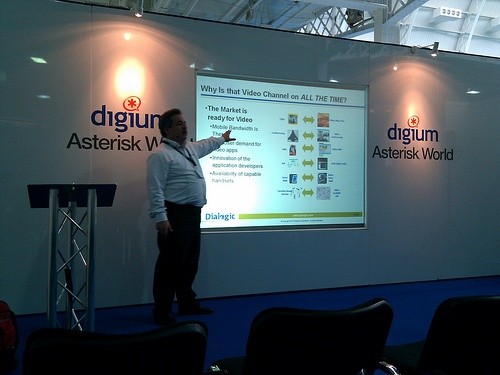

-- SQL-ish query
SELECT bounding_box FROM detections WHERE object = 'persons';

[146,109,236,327]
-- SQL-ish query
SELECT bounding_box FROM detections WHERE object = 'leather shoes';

[178,308,213,315]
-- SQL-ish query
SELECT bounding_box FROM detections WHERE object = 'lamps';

[412,42,439,57]
[134,1,144,18]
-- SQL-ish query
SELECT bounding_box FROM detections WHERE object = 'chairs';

[23,319,208,375]
[418,296,500,375]
[208,297,401,375]
